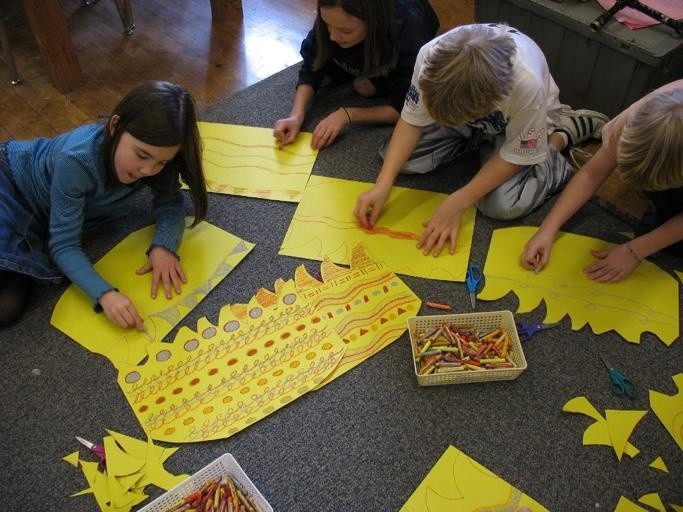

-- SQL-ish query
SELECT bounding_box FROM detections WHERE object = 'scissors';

[598,351,637,400]
[515,321,561,342]
[75,436,106,471]
[466,261,483,309]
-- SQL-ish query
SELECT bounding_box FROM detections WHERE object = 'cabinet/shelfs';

[501,0,682,119]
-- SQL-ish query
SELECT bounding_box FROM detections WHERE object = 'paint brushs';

[437,370,450,373]
[422,365,435,375]
[442,352,464,364]
[416,350,442,357]
[507,357,518,367]
[493,345,501,358]
[416,327,440,346]
[535,254,539,274]
[247,495,262,512]
[227,502,234,512]
[431,328,443,341]
[419,354,443,375]
[439,367,464,371]
[366,218,372,230]
[236,490,255,512]
[416,340,432,361]
[479,329,500,342]
[432,346,459,352]
[228,497,233,502]
[219,484,227,512]
[484,343,492,354]
[457,329,485,371]
[227,472,249,497]
[226,477,237,497]
[279,132,291,150]
[502,329,512,346]
[478,328,496,338]
[486,363,512,367]
[496,333,507,346]
[436,362,461,366]
[445,325,456,344]
[142,325,146,332]
[159,499,186,512]
[184,481,219,512]
[426,301,453,311]
[450,331,456,338]
[173,503,191,512]
[502,336,509,358]
[480,358,505,363]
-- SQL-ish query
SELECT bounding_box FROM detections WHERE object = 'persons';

[0,82,210,333]
[356,23,609,257]
[518,79,683,284]
[272,1,440,151]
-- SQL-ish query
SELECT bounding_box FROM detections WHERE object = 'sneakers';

[554,104,610,170]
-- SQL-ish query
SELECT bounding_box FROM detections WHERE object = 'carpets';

[1,58,683,512]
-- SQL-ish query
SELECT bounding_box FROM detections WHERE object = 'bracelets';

[340,105,352,125]
[626,241,642,263]
